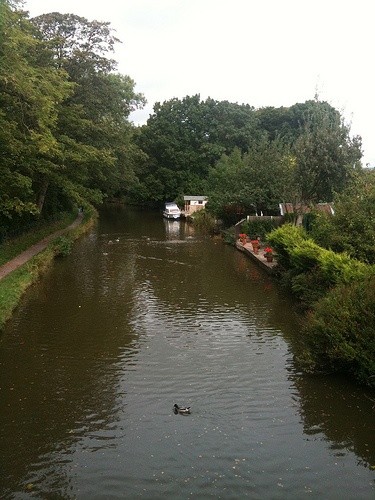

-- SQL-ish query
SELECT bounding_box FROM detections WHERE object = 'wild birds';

[173,404,191,413]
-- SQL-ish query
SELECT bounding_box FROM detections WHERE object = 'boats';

[163,203,183,221]
